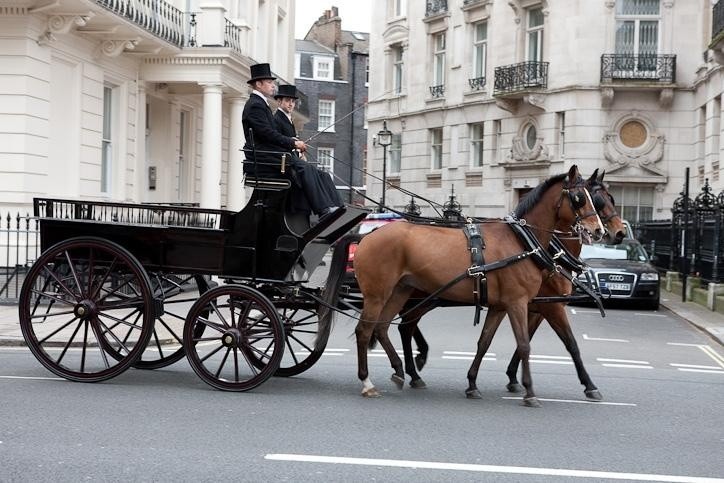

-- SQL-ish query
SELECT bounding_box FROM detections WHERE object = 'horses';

[312,163,607,410]
[367,166,630,401]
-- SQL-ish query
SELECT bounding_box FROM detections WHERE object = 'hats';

[246,62,277,83]
[273,84,299,100]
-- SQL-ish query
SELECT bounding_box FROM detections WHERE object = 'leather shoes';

[320,206,340,219]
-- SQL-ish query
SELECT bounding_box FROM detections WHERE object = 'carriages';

[18,164,625,412]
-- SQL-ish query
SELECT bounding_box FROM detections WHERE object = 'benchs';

[238,127,293,192]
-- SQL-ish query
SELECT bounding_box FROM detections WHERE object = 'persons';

[241,60,347,221]
[273,83,347,209]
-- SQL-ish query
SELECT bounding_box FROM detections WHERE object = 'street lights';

[377,118,395,210]
